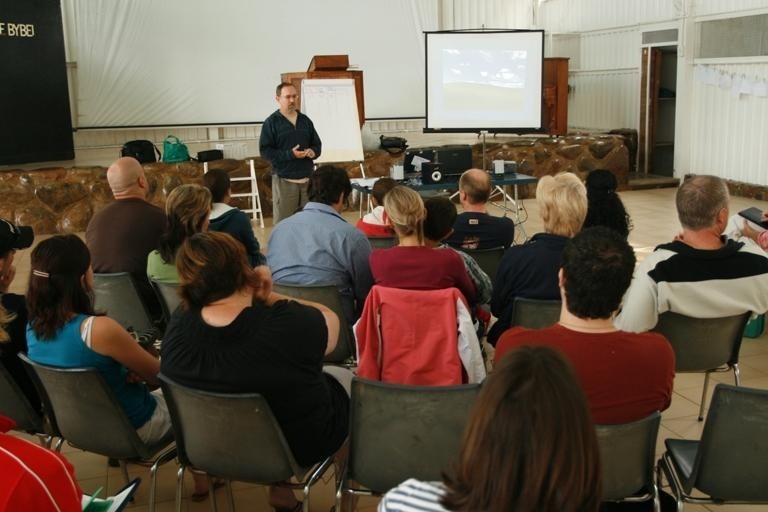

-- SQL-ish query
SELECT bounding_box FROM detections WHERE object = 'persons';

[614,176,768,333]
[583,170,632,241]
[27,233,171,445]
[162,233,352,511]
[423,197,493,337]
[729,209,768,258]
[493,228,675,425]
[260,82,321,225]
[0,218,54,438]
[377,346,601,511]
[203,169,266,269]
[486,171,589,349]
[86,158,167,323]
[445,169,514,249]
[267,166,372,323]
[356,179,396,237]
[147,185,211,282]
[369,186,487,359]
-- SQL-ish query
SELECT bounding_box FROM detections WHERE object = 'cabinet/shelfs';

[544,56,570,136]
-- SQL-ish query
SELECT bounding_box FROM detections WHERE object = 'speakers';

[421,162,445,184]
[438,144,472,183]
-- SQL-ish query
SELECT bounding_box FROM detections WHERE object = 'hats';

[1,218,34,252]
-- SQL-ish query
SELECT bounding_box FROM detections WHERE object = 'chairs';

[366,236,394,250]
[657,383,768,512]
[90,272,151,335]
[334,377,481,512]
[510,296,562,329]
[271,281,358,370]
[150,278,184,325]
[457,246,505,278]
[587,409,662,512]
[155,373,334,512]
[0,364,54,448]
[17,352,177,512]
[661,309,753,421]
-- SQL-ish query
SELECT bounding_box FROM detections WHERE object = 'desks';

[350,173,538,242]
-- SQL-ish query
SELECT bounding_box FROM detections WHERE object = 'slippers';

[191,476,230,502]
[276,500,301,510]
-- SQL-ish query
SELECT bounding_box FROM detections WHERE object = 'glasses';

[278,94,299,100]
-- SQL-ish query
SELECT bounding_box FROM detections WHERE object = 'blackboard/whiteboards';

[301,80,364,163]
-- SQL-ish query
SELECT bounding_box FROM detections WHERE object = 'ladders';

[204,159,265,227]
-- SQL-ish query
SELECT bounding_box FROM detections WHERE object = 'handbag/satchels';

[162,136,190,163]
[119,140,162,165]
[381,135,407,154]
